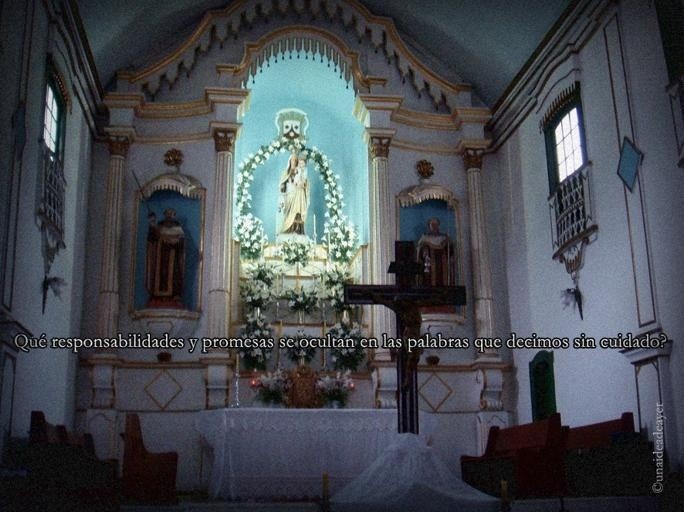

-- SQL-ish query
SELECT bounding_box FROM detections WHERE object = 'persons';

[278,153,311,235]
[415,216,456,313]
[293,159,308,186]
[143,207,187,309]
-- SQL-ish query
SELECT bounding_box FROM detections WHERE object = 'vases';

[235,139,367,408]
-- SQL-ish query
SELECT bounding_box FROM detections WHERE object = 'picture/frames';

[199,408,401,500]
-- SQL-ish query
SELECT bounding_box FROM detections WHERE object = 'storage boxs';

[458,410,658,499]
[28,408,178,510]
